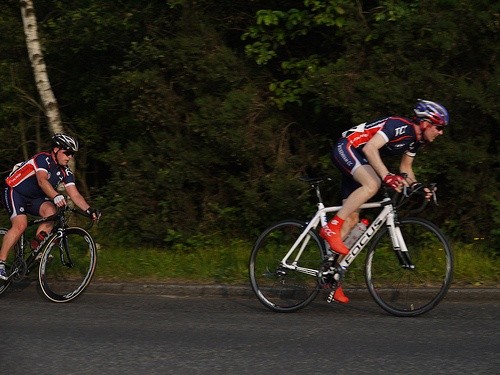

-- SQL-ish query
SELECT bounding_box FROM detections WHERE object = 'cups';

[29,231,48,251]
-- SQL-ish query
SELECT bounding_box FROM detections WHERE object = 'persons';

[0,134,99,281]
[318,98,449,304]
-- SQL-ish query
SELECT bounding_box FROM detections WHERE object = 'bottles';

[343,220,368,249]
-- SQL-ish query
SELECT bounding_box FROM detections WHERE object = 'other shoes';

[323,279,349,303]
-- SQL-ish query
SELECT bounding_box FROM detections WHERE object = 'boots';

[319,214,349,255]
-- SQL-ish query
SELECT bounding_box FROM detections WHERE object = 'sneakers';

[0,259,9,281]
[35,251,54,261]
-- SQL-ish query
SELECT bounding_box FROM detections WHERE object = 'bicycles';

[1,194,100,304]
[248,175,455,317]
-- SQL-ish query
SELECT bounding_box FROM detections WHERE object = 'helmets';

[51,134,79,152]
[414,98,449,126]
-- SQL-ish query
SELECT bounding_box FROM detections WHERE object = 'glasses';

[435,127,447,131]
[62,150,74,158]
